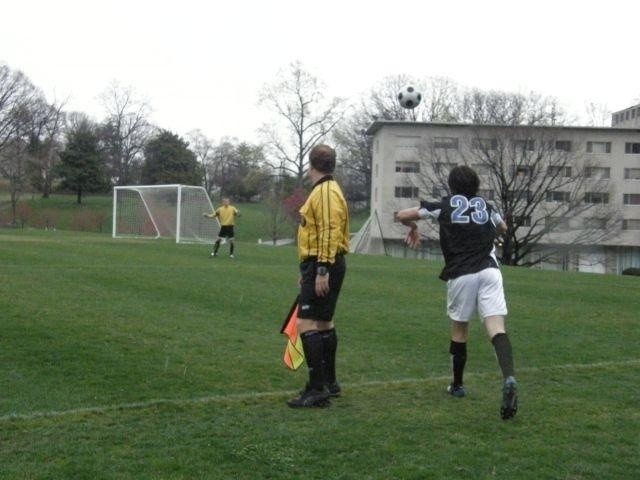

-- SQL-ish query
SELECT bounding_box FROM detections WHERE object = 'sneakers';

[285,383,340,407]
[445,383,468,399]
[499,375,519,419]
[210,253,235,259]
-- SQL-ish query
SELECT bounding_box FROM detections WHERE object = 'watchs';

[316,266,329,275]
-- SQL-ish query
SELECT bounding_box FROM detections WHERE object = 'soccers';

[398,85,421,108]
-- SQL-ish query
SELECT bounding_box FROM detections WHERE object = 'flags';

[280,296,306,372]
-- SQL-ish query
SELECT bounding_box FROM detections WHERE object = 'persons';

[284,144,351,408]
[396,165,519,421]
[201,195,242,258]
